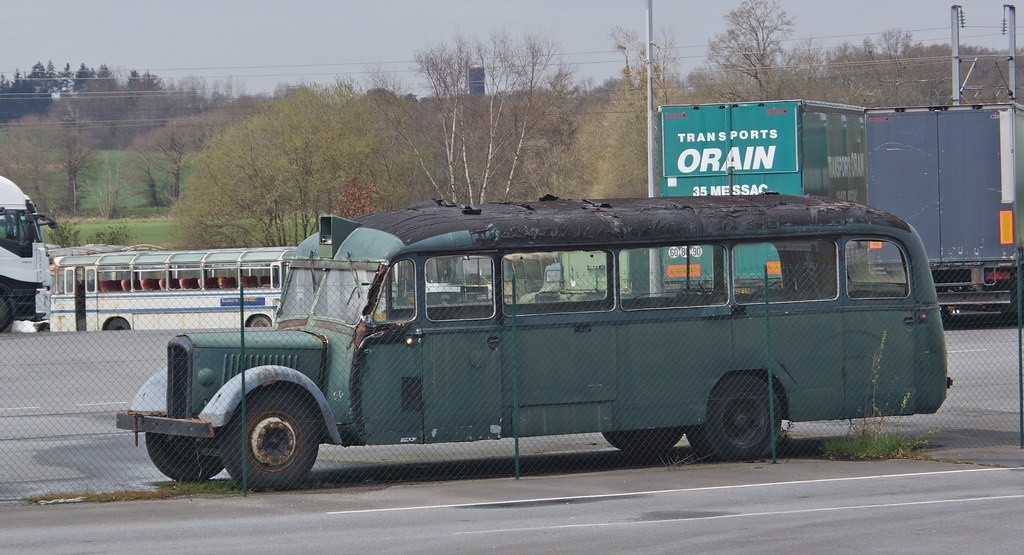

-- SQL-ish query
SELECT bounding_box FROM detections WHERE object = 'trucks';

[0,176,58,334]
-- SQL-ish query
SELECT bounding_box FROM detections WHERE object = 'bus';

[115,195,952,490]
[46,246,492,330]
[462,251,561,304]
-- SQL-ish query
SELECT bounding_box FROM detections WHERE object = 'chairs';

[62,278,101,297]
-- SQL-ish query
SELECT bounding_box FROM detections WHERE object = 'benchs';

[179,278,198,289]
[260,276,278,285]
[158,279,180,290]
[121,279,142,291]
[197,277,220,289]
[240,275,257,288]
[218,277,236,288]
[114,280,122,290]
[99,280,117,292]
[140,279,161,290]
